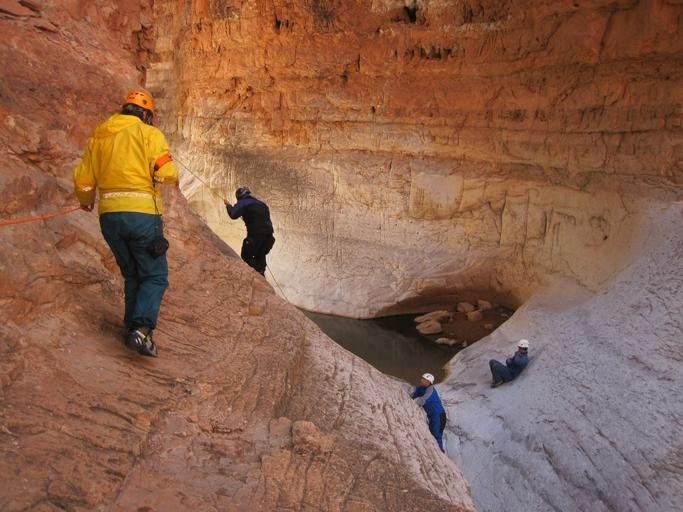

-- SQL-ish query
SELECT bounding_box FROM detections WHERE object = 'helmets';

[422,372,435,384]
[122,91,155,115]
[517,339,529,348]
[236,187,250,199]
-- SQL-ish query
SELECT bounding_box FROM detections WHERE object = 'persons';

[487,339,530,387]
[223,186,275,274]
[409,373,448,453]
[72,92,178,357]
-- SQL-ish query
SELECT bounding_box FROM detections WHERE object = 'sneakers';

[126,329,157,356]
[492,380,503,388]
